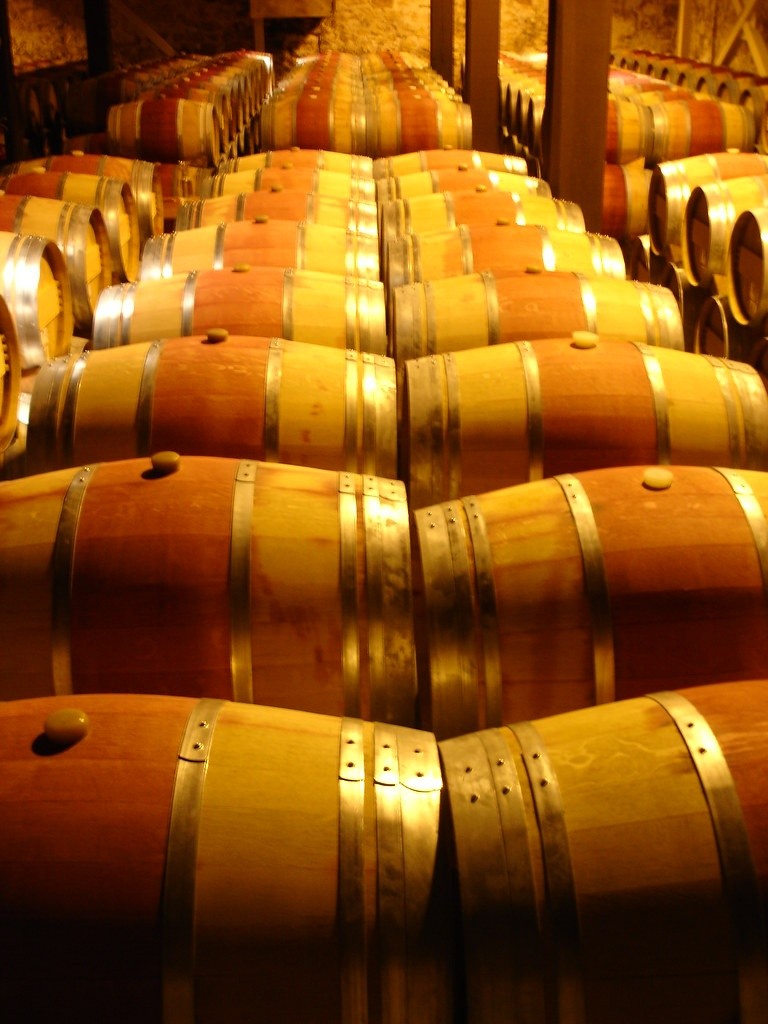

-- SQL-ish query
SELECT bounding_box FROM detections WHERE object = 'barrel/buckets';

[0,47,768,1024]
